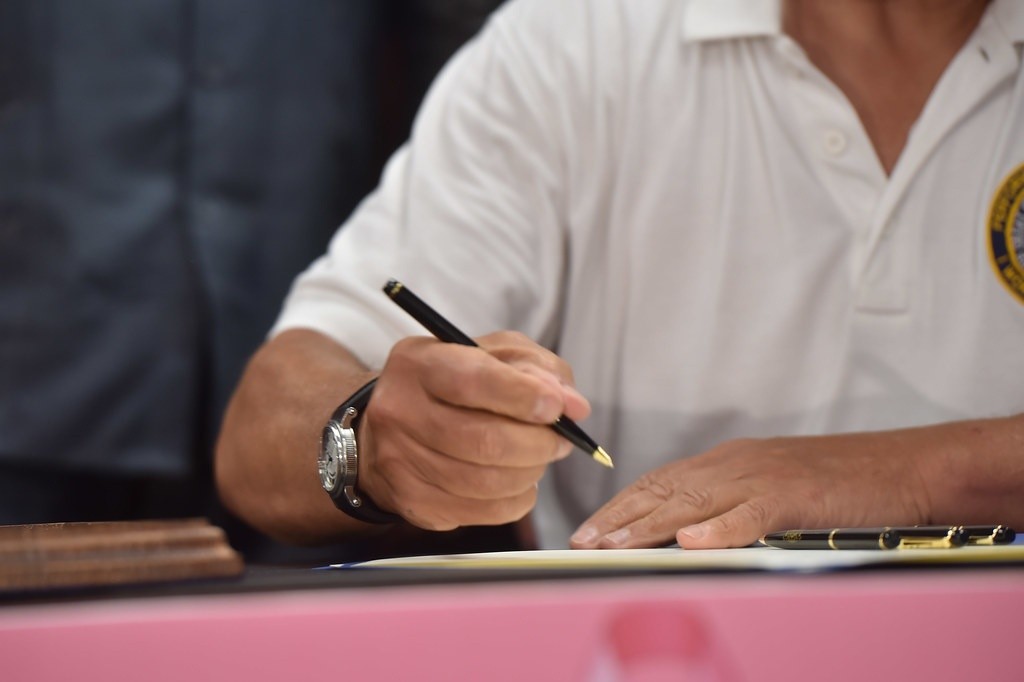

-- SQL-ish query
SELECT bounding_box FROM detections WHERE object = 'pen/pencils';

[383,281,614,469]
[759,524,1016,549]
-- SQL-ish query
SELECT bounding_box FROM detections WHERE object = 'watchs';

[317,376,403,526]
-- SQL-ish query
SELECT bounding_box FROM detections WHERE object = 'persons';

[214,0,1024,552]
